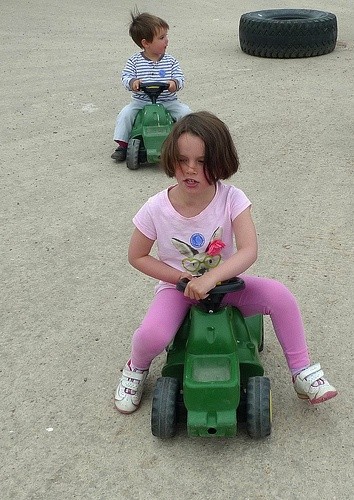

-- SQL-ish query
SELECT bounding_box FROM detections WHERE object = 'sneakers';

[293,363,339,405]
[110,147,127,162]
[114,356,149,414]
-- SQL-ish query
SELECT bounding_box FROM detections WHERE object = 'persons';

[111,11,193,161]
[113,110,339,414]
